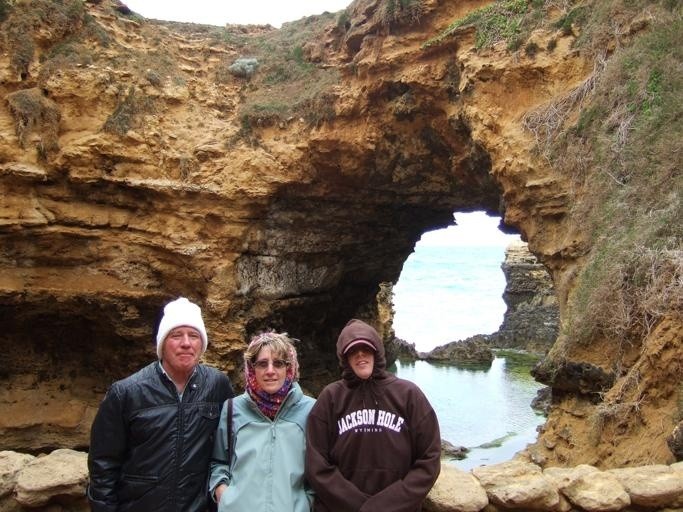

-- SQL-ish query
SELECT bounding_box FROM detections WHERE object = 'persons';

[87,297,236,511]
[304,318,442,512]
[207,331,317,511]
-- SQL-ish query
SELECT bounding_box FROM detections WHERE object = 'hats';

[156,297,208,360]
[343,339,377,355]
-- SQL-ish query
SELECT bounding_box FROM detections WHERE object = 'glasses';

[347,344,376,355]
[253,359,288,369]
